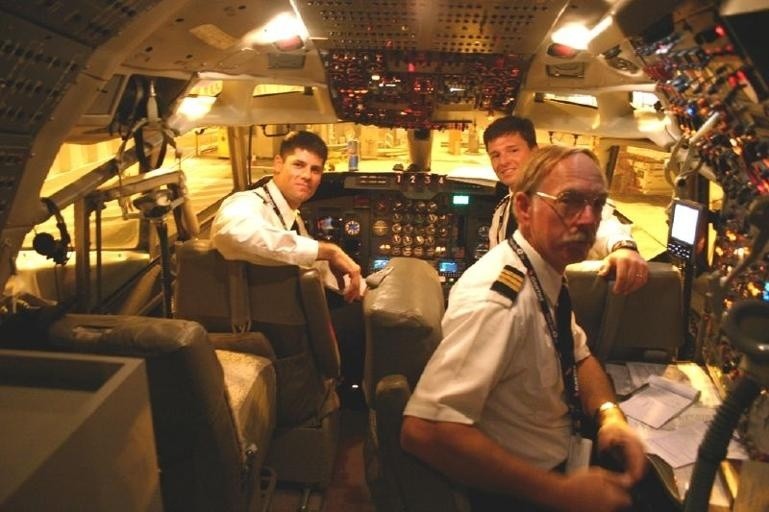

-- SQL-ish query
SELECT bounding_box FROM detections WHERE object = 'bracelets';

[593,402,628,434]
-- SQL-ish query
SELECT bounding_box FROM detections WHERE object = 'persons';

[484,116,650,296]
[400,146,648,512]
[209,129,366,411]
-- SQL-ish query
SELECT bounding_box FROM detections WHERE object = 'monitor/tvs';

[666,198,706,264]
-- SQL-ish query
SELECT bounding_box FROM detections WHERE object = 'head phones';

[33,197,71,266]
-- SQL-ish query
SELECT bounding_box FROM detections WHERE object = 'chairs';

[561,256,686,361]
[358,251,476,512]
[41,301,281,512]
[161,228,343,491]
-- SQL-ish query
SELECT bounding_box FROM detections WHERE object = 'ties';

[289,214,302,238]
[554,274,586,436]
[503,192,520,240]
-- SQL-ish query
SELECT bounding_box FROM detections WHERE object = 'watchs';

[612,240,639,253]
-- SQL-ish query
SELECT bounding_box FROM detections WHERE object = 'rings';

[636,273,644,279]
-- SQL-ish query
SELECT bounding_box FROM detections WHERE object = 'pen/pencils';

[622,382,649,401]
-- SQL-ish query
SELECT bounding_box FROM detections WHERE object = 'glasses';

[528,188,615,216]
